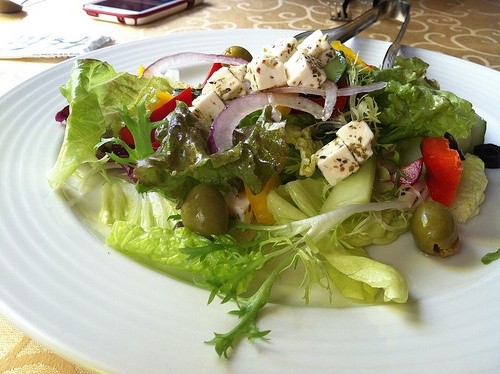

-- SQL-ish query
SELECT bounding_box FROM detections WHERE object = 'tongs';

[294,0,412,71]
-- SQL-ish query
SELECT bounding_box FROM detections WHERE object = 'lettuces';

[53,54,488,304]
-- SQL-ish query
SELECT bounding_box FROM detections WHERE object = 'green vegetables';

[167,200,413,361]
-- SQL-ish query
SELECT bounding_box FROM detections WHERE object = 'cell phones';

[82,0,203,25]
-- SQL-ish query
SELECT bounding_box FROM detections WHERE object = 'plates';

[1,26,500,371]
